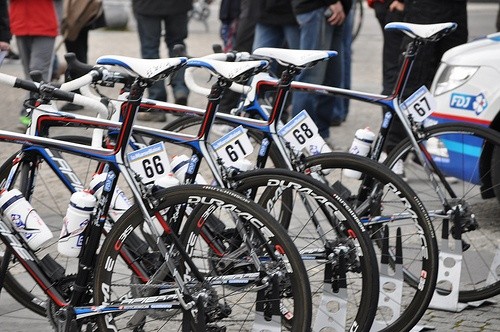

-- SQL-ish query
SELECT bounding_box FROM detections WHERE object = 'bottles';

[170,154,207,185]
[342,127,374,179]
[89,172,132,223]
[211,158,251,187]
[154,173,179,190]
[306,134,334,174]
[0,188,54,251]
[57,188,96,258]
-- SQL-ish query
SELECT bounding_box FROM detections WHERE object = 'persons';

[206,0,355,152]
[368,0,468,184]
[133,0,192,121]
[0,0,20,59]
[9,0,107,132]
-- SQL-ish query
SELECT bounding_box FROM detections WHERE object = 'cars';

[410,33,500,202]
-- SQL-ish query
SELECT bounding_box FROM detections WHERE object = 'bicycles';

[0,20,500,332]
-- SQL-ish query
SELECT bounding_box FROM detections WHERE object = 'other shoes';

[322,116,347,151]
[136,101,167,120]
[173,90,188,117]
[377,151,406,176]
[19,115,34,132]
